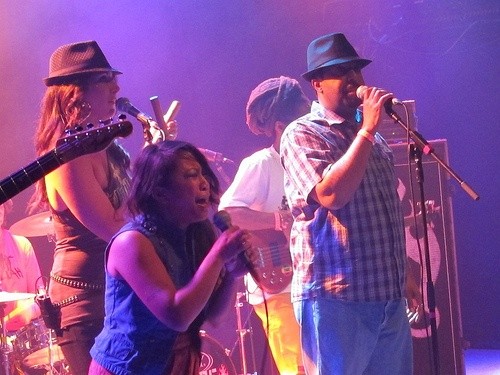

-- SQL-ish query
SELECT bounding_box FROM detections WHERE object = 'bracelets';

[273,210,283,231]
[357,128,374,144]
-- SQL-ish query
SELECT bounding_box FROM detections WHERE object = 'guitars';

[243,200,442,294]
[0,113,134,206]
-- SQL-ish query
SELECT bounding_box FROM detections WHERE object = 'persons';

[0,33,415,375]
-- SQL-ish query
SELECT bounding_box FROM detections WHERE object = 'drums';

[15,316,67,368]
[199,331,237,375]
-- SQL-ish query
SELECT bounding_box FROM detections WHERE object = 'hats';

[300,33,373,82]
[43,40,123,87]
[246,76,303,138]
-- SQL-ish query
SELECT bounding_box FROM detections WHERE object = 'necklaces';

[144,221,176,277]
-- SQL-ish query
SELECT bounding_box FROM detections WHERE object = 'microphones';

[213,210,262,284]
[115,97,161,131]
[356,85,404,105]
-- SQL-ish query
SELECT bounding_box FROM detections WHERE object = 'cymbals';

[9,211,55,237]
[0,291,36,303]
